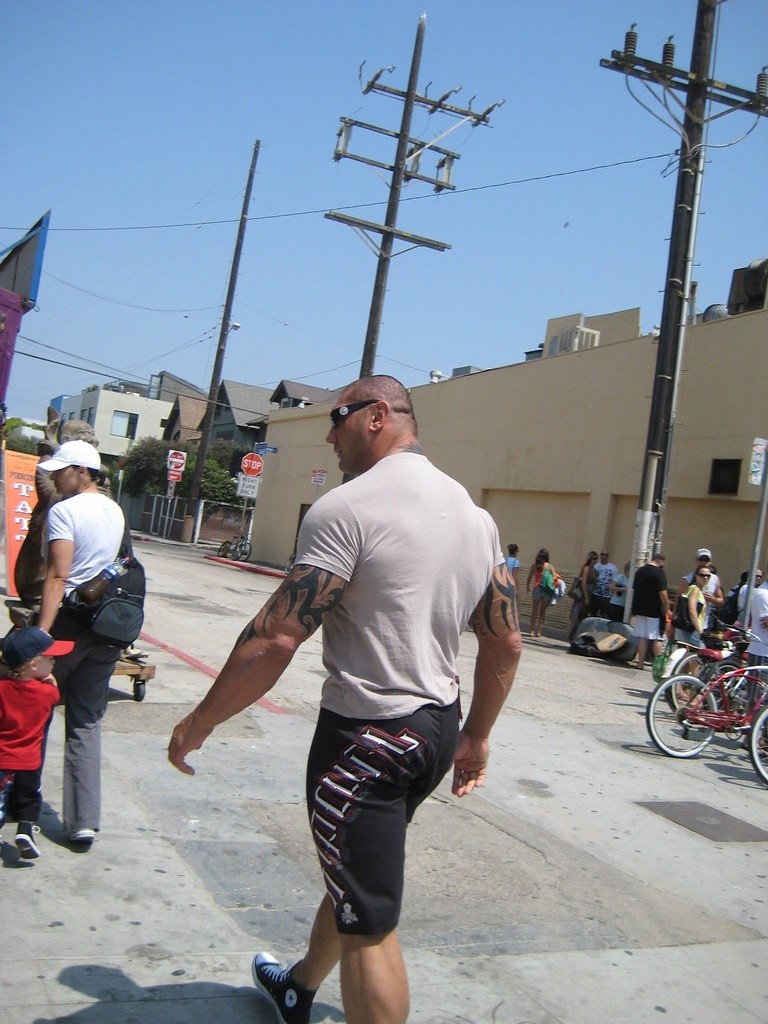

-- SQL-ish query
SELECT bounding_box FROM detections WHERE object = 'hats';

[695,548,712,559]
[37,439,102,473]
[3,625,74,669]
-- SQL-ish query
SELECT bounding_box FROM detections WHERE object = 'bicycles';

[645,612,768,787]
[218,535,253,561]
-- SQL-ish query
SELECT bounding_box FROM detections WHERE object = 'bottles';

[82,557,129,603]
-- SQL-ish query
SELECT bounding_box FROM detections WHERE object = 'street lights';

[181,322,240,544]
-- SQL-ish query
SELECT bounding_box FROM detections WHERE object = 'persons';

[169,375,522,1024]
[8,439,134,840]
[504,544,519,598]
[14,406,114,613]
[569,548,630,644]
[0,627,74,858]
[666,548,768,758]
[527,549,558,636]
[631,554,677,670]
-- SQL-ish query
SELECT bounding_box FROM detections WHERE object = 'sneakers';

[251,950,317,1024]
[14,821,40,860]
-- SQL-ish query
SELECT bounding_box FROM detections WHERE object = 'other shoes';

[70,829,96,842]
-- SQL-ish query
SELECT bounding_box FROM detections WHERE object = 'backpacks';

[718,587,744,625]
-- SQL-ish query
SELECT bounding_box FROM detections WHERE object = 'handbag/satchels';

[59,501,147,651]
[540,564,555,594]
[652,640,673,684]
[670,596,703,632]
[566,577,597,603]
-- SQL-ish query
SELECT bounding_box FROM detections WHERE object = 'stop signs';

[167,450,188,472]
[241,454,265,476]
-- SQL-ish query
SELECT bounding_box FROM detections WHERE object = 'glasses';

[697,573,711,578]
[600,553,609,556]
[329,399,380,428]
[698,557,709,561]
[591,557,598,560]
